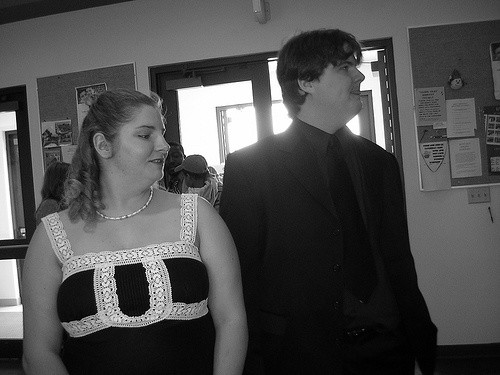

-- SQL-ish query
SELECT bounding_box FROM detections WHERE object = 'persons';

[22,88,248,375]
[174,154,223,212]
[218,28,439,375]
[34,161,73,227]
[447,69,464,89]
[164,142,186,194]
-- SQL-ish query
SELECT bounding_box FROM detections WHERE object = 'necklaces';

[96,186,153,220]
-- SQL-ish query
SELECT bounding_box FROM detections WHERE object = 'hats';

[182,155,208,174]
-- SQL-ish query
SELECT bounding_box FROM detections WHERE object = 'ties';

[326,135,371,301]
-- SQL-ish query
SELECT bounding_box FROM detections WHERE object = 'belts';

[342,327,380,345]
